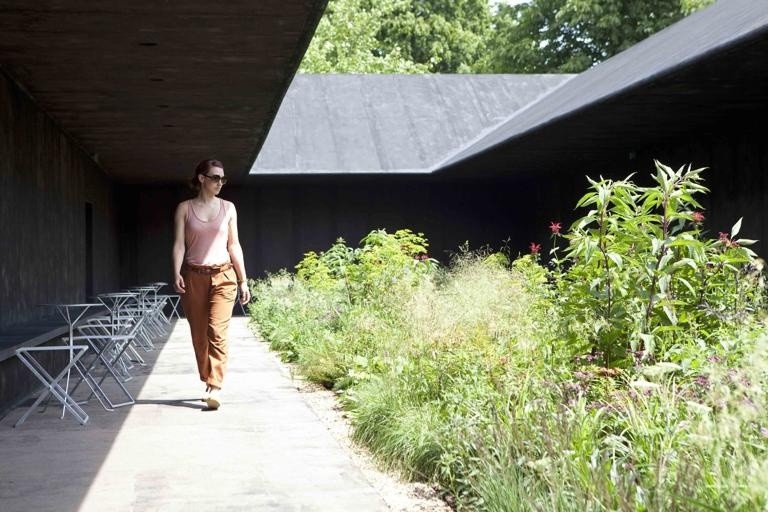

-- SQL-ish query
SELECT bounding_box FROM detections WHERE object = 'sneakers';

[202,386,221,408]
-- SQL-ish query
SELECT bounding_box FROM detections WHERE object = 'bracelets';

[239,278,248,284]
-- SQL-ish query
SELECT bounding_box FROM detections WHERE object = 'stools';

[13,282,181,428]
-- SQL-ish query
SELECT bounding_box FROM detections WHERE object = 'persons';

[170,159,250,410]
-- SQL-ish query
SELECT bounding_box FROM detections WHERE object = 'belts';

[187,263,233,274]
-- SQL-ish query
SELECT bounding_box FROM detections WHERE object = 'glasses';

[202,173,228,184]
